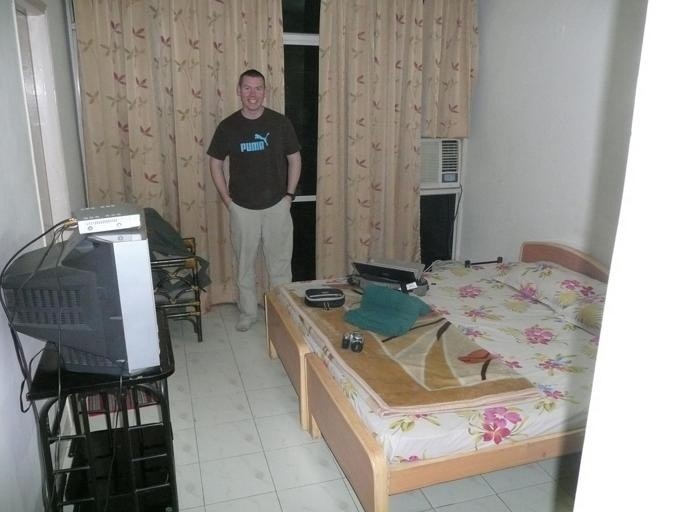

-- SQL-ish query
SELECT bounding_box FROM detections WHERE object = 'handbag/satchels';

[305,287,346,310]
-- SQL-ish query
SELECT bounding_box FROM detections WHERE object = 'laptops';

[350,256,425,282]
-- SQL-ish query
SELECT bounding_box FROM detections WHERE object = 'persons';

[207,69,303,331]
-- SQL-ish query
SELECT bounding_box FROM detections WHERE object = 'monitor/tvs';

[1,209,162,376]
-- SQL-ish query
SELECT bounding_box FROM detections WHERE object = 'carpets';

[87,381,159,416]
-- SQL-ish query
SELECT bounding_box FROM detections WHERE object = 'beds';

[264,243,610,512]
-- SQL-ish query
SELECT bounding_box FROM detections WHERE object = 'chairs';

[149,237,202,343]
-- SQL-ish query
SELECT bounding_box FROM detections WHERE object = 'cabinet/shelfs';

[26,308,178,512]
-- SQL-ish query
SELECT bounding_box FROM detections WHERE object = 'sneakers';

[235,318,257,332]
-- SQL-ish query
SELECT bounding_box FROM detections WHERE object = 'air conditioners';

[418,137,462,189]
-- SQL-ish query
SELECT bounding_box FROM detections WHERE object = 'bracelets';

[284,193,296,200]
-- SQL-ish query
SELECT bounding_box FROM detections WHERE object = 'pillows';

[494,260,607,313]
[557,292,607,337]
